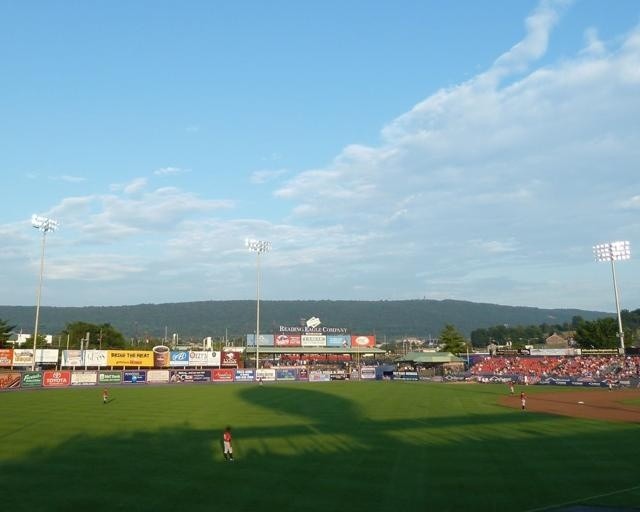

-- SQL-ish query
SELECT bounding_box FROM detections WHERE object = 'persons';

[222,426,236,463]
[258,376,264,390]
[468,355,640,393]
[101,386,110,404]
[508,381,515,395]
[520,391,529,410]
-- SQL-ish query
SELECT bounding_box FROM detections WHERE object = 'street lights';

[30,213,59,371]
[245,238,271,369]
[592,240,631,351]
